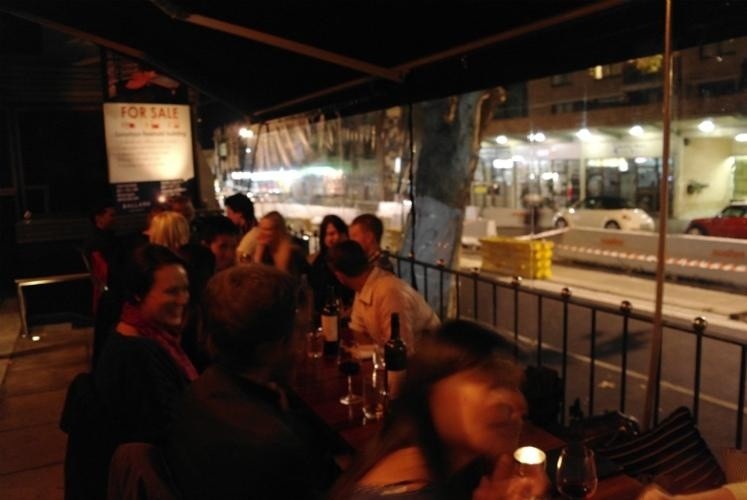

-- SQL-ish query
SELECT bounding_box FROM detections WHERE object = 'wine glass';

[336,338,365,406]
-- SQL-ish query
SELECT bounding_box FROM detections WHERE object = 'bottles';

[384,312,411,406]
[292,272,316,346]
[320,278,341,360]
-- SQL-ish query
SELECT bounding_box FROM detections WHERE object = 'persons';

[82,191,746,500]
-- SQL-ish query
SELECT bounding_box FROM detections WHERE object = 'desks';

[260,304,641,500]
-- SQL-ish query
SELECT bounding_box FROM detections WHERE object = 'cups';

[556,444,598,500]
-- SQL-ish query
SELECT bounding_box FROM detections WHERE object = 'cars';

[551,196,655,231]
[685,204,747,239]
